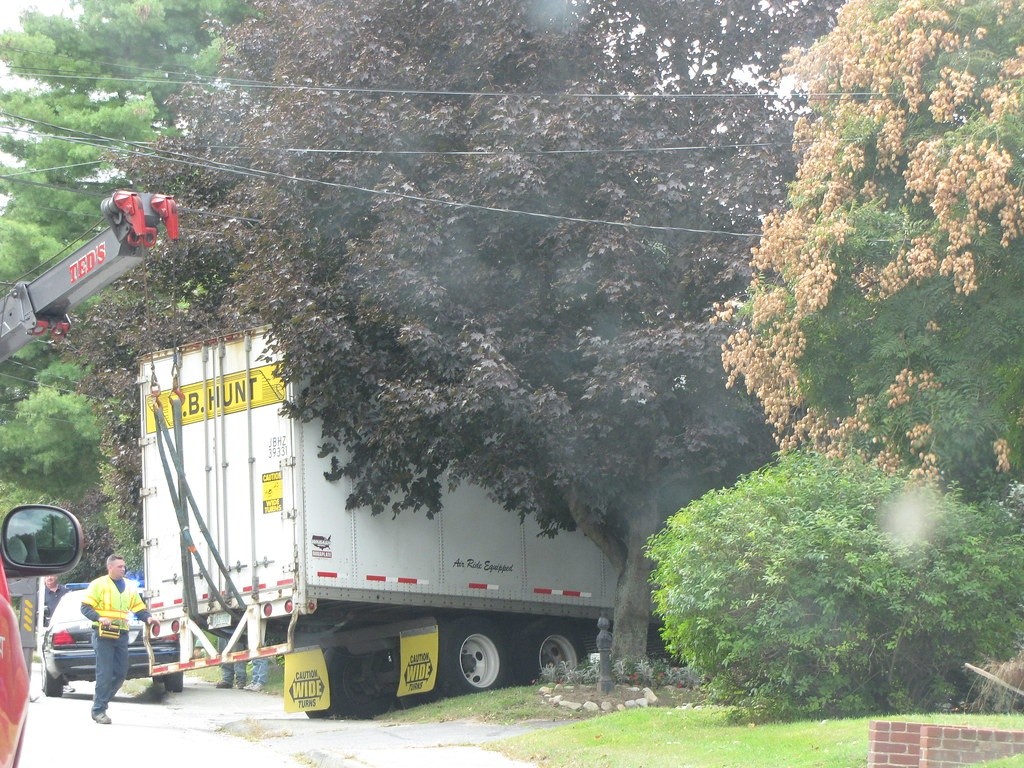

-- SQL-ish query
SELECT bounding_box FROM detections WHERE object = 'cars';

[0,503,83,768]
[37,582,185,698]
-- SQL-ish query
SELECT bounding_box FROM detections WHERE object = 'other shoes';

[242,682,256,690]
[216,682,232,688]
[235,681,245,688]
[250,683,263,691]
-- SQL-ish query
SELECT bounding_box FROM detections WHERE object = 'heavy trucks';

[133,321,671,717]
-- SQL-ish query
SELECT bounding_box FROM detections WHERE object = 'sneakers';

[91,709,111,724]
[63,683,75,692]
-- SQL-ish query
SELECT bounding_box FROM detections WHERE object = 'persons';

[44,575,69,616]
[216,637,246,688]
[80,554,160,724]
[244,656,270,692]
